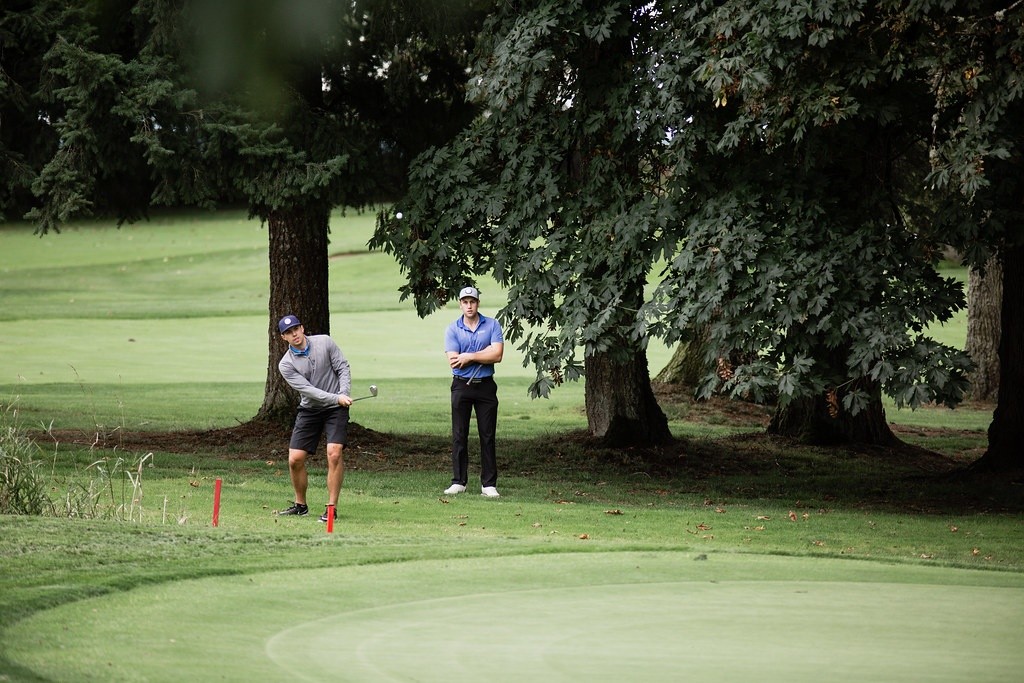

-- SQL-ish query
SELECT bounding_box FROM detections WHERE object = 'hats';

[458,287,479,301]
[279,315,301,333]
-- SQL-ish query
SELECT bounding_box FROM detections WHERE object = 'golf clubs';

[350,386,378,405]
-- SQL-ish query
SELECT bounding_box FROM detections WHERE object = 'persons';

[445,287,503,497]
[278,315,351,523]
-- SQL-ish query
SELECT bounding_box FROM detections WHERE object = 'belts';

[454,375,492,383]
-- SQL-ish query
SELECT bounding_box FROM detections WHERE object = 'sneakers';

[481,485,500,498]
[279,500,308,516]
[444,484,467,495]
[322,504,338,521]
